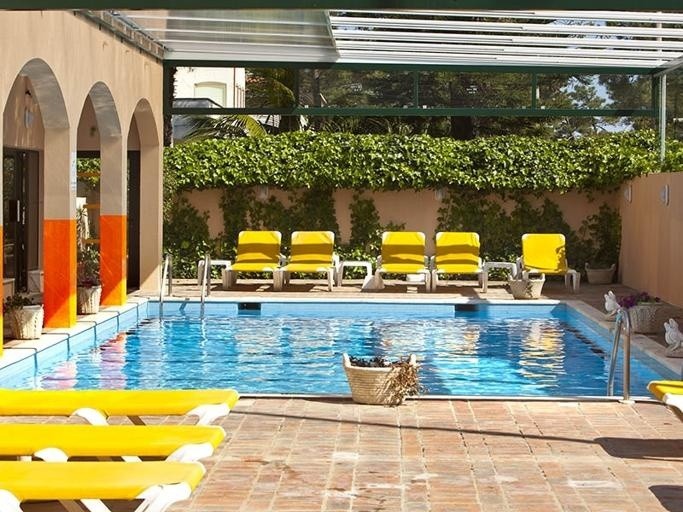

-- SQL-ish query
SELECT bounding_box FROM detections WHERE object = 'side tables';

[337,261,372,289]
[484,261,517,295]
[197,260,231,289]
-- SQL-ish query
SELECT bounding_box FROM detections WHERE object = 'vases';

[629,305,679,333]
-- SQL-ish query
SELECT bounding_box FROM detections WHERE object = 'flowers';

[616,290,665,303]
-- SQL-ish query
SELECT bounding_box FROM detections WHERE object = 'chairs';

[226,230,281,290]
[278,231,336,291]
[375,231,431,292]
[521,233,581,294]
[433,231,483,293]
[645,380,682,416]
[0,388,241,508]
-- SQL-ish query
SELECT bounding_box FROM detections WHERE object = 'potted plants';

[2,290,43,341]
[77,240,102,314]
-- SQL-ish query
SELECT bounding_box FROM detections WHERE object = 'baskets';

[614,291,668,335]
[7,302,44,341]
[78,276,103,315]
